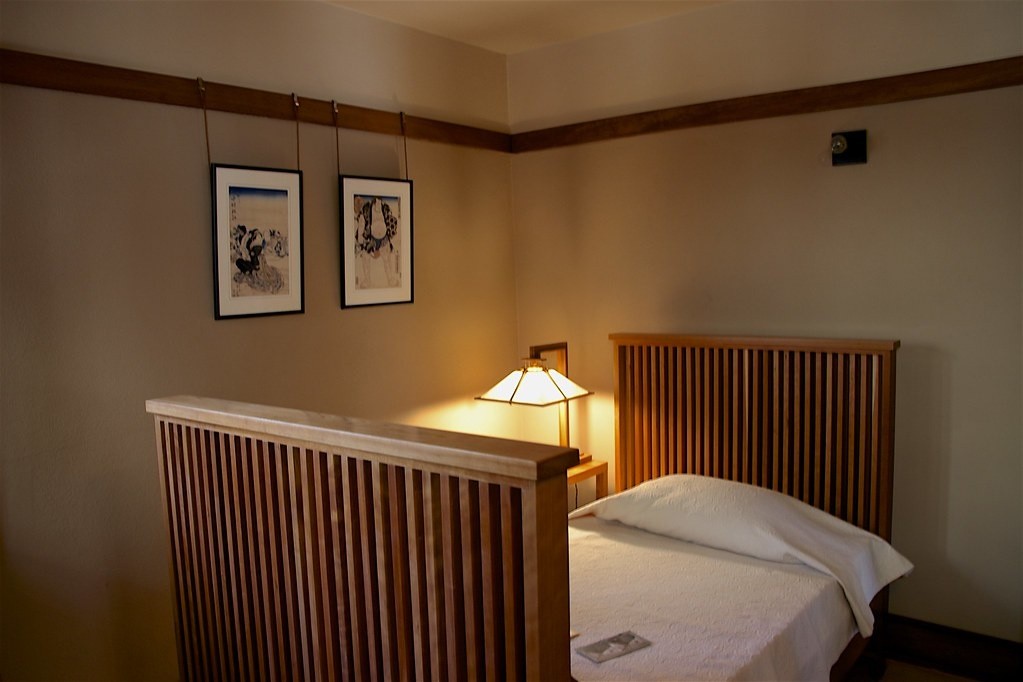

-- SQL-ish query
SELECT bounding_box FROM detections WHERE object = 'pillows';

[620,475,816,562]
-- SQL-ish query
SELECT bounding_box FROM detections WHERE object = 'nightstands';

[566,460,609,502]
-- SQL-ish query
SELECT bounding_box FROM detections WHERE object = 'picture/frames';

[210,161,305,320]
[341,176,414,309]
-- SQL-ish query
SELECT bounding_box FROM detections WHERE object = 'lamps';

[474,343,595,465]
[829,131,868,168]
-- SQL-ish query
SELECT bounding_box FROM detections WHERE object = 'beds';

[145,333,902,681]
